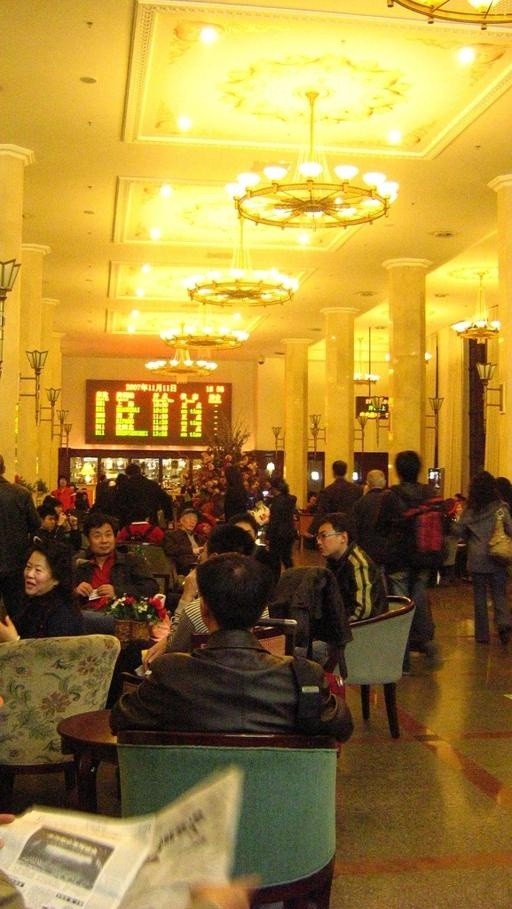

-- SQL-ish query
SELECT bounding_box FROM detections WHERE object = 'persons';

[162,509,207,576]
[103,464,173,517]
[266,477,298,569]
[108,552,354,743]
[105,523,268,711]
[29,505,82,556]
[351,470,392,555]
[450,472,512,644]
[0,542,83,643]
[70,481,95,513]
[302,493,318,514]
[227,513,280,588]
[224,465,250,522]
[52,476,76,513]
[114,505,164,543]
[0,456,42,635]
[366,450,457,677]
[71,514,159,613]
[313,513,388,624]
[306,461,364,539]
[43,495,72,538]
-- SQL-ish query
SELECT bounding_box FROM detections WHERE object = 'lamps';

[20,349,49,399]
[40,386,61,424]
[353,373,379,385]
[273,425,285,451]
[60,422,73,453]
[179,221,300,310]
[158,312,249,348]
[474,361,505,415]
[51,408,70,438]
[227,88,402,234]
[429,395,444,416]
[0,256,21,306]
[308,413,327,443]
[354,413,369,441]
[452,320,499,345]
[144,348,215,377]
[374,393,390,430]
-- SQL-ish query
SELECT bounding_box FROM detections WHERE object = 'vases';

[131,619,152,645]
[113,617,131,652]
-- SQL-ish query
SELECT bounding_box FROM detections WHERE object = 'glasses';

[316,532,341,541]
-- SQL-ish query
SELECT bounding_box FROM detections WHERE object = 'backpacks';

[393,484,458,571]
[125,524,156,542]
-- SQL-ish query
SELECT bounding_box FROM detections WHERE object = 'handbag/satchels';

[489,504,511,561]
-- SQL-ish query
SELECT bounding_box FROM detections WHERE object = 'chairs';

[333,594,417,740]
[254,617,300,657]
[109,731,344,888]
[1,632,121,816]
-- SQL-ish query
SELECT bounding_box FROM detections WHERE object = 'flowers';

[132,592,172,627]
[104,592,141,621]
